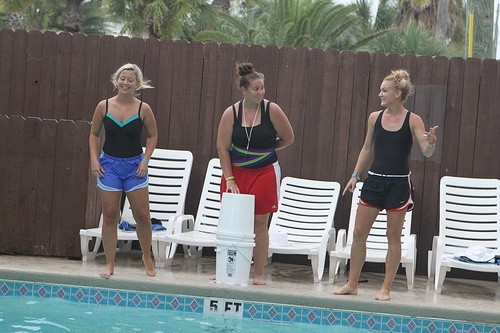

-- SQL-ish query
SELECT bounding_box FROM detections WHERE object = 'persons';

[209,63,295,286]
[89,64,158,279]
[332,69,439,301]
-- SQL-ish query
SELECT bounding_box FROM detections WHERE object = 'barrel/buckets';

[215,240,256,286]
[214,192,256,252]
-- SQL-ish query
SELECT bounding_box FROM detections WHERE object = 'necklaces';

[243,101,260,149]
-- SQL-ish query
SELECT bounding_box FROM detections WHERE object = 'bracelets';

[226,177,234,180]
[352,174,358,178]
[145,160,149,163]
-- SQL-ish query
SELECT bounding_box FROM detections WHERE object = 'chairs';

[78,146,195,270]
[267,177,342,284]
[328,182,418,292]
[156,159,281,268]
[427,177,500,294]
